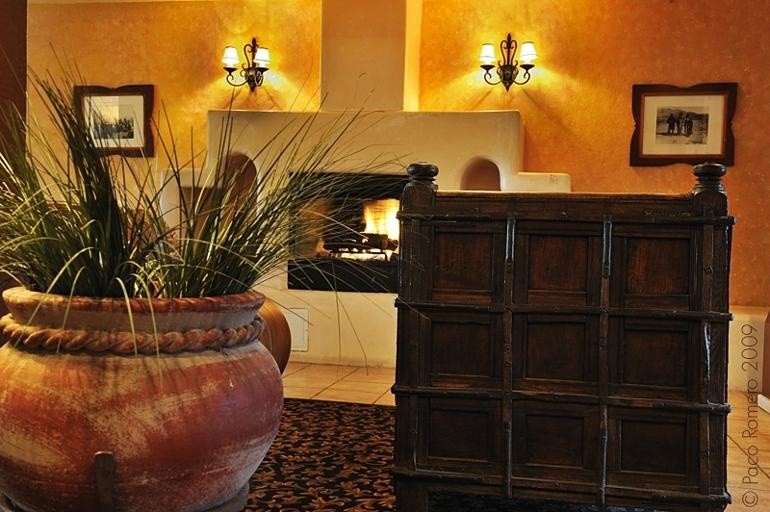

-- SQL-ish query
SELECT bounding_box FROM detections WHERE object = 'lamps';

[222,38,271,97]
[479,34,538,92]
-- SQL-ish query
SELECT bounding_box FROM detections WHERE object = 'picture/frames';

[71,83,154,158]
[630,82,738,167]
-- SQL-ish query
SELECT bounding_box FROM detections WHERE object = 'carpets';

[244,398,396,510]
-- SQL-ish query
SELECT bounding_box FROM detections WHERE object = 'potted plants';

[0,43,388,512]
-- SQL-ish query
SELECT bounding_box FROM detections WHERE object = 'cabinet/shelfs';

[391,158,736,512]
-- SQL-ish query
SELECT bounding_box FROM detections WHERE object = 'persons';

[666,112,693,137]
